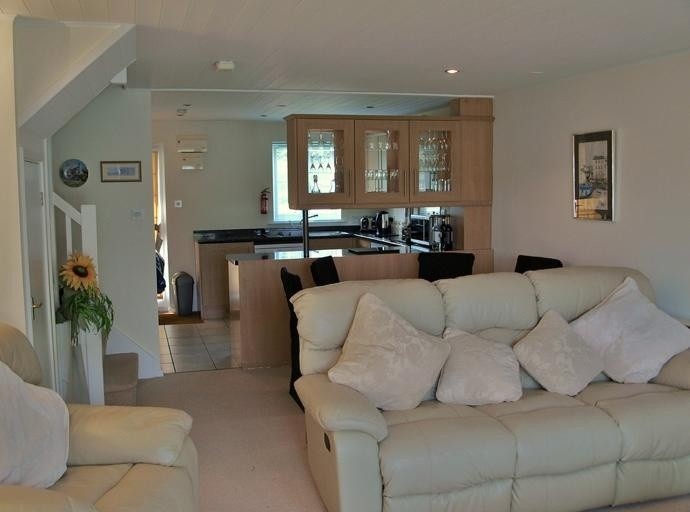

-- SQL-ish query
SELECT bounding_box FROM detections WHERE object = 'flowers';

[54,250,115,346]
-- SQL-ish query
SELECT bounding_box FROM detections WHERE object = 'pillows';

[569,276,690,383]
[512,309,604,396]
[328,292,450,412]
[435,326,523,406]
[0,359,68,488]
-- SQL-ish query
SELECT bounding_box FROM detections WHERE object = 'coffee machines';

[428,213,454,251]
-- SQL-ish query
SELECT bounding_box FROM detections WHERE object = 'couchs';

[287,266,690,512]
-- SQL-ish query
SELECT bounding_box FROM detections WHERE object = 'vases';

[57,343,92,404]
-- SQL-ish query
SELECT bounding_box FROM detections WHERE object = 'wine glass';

[417,129,453,193]
[306,128,345,173]
[363,130,400,193]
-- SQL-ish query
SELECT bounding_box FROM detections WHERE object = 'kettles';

[373,210,393,235]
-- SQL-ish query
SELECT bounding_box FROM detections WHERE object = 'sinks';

[309,230,349,237]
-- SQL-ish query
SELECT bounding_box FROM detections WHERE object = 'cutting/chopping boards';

[348,247,400,255]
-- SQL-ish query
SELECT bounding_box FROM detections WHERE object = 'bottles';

[330,178,342,193]
[312,174,320,194]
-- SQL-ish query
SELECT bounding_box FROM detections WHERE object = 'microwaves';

[409,215,430,246]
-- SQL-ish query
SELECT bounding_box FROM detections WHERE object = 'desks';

[225,246,494,371]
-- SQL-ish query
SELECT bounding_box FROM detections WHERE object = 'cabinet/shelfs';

[194,241,253,320]
[283,114,493,209]
[308,238,355,248]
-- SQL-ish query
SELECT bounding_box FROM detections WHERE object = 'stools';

[102,353,139,406]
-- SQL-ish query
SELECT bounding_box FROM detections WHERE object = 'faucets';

[300,215,318,225]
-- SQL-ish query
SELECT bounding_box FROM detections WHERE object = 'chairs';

[515,254,562,274]
[280,266,305,413]
[418,252,475,282]
[310,256,340,286]
[1,321,200,512]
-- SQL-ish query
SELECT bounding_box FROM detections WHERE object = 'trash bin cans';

[171,271,194,316]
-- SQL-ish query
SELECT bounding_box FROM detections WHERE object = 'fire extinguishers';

[260,187,271,214]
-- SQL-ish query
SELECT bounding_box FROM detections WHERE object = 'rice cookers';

[359,215,377,231]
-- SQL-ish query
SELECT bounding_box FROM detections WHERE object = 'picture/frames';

[573,128,614,221]
